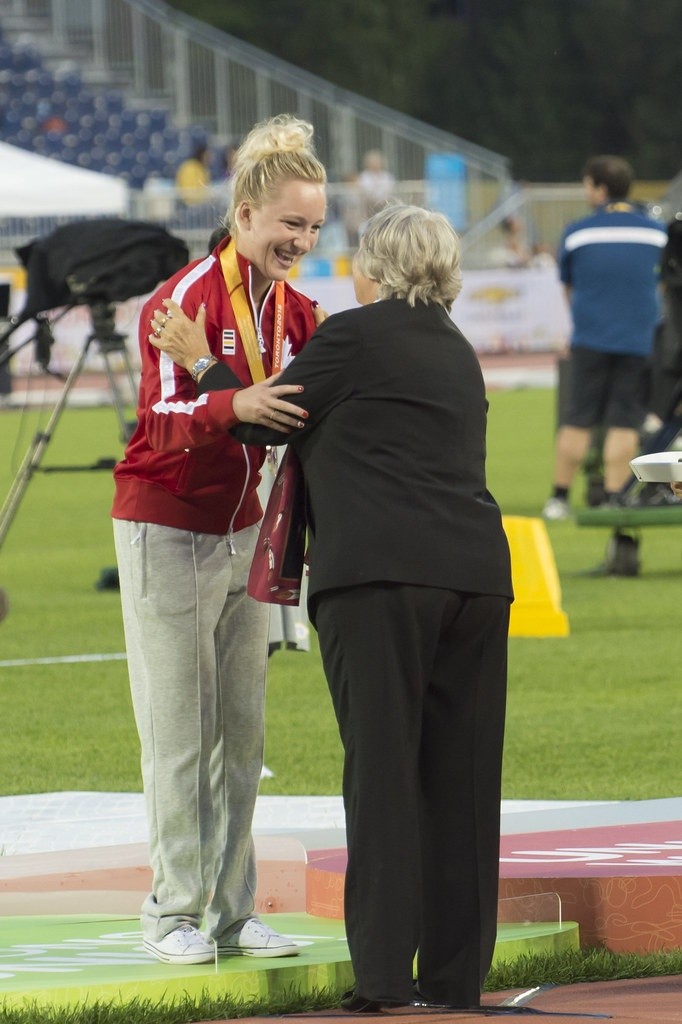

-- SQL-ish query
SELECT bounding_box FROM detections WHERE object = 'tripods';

[0,301,139,548]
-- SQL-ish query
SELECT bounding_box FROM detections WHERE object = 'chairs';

[1,38,244,238]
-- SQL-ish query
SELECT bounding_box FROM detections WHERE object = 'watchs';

[192,355,218,382]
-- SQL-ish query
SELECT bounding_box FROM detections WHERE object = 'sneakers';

[218,918,301,958]
[141,924,215,965]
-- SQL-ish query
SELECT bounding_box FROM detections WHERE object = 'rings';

[161,317,170,327]
[154,326,164,335]
[270,410,276,419]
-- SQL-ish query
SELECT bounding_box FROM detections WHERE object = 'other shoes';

[640,485,681,507]
[590,487,619,507]
[542,498,568,521]
[343,992,411,1013]
[411,983,481,1009]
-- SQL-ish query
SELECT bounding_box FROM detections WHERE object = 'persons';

[542,155,669,525]
[149,204,515,1014]
[112,114,327,963]
[167,142,401,253]
[584,203,682,508]
